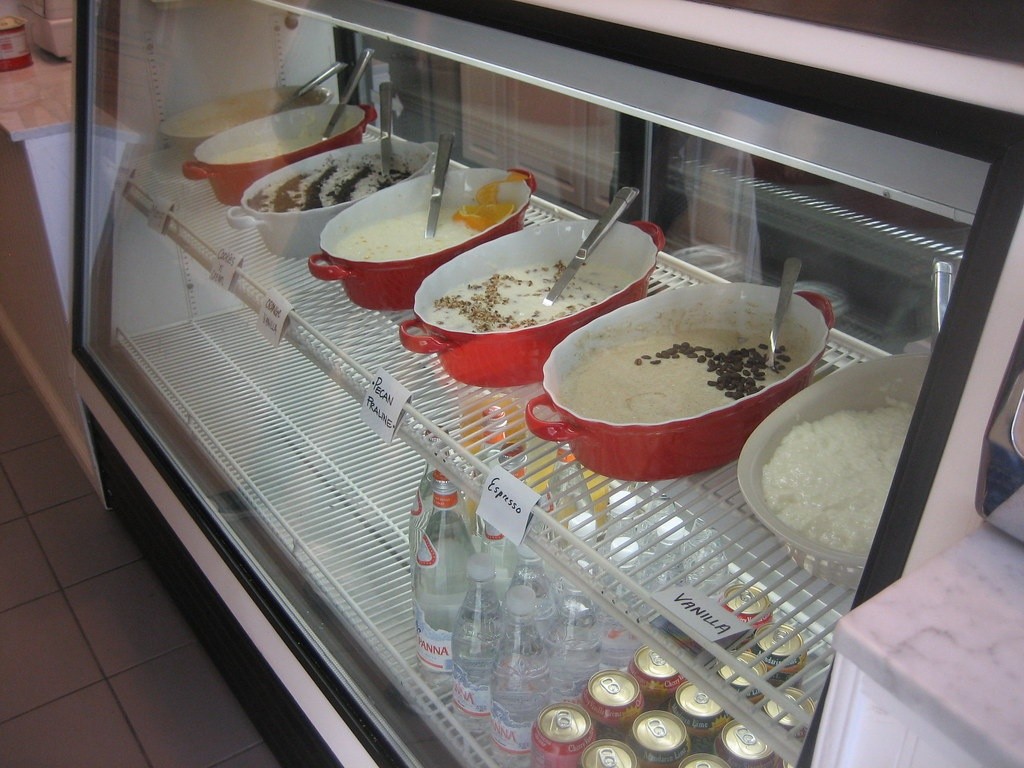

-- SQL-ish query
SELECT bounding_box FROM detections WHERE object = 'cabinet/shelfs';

[1,45,126,511]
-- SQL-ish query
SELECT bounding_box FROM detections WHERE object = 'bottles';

[409,406,728,768]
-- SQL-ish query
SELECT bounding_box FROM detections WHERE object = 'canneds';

[530,584,816,768]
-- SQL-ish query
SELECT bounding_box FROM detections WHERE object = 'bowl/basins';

[737,353,932,592]
[308,168,536,312]
[157,83,334,162]
[524,282,835,481]
[226,140,440,262]
[399,220,666,387]
